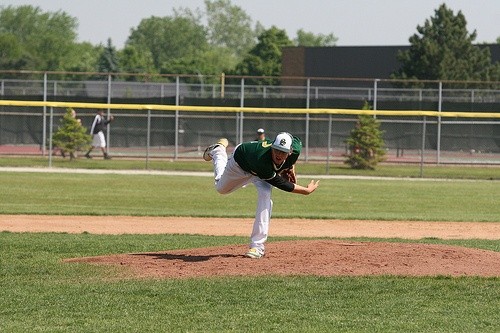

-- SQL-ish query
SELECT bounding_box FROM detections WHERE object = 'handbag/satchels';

[94,116,103,134]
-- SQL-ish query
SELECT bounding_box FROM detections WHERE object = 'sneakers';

[203,137,229,161]
[244,248,265,259]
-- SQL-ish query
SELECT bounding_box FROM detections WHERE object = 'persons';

[86,109,114,159]
[202,129,320,259]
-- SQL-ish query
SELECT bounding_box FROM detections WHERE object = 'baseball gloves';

[280,169,297,184]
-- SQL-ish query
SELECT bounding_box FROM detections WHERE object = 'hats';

[271,131,294,152]
[257,128,264,134]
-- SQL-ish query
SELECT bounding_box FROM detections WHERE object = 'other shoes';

[84,153,91,158]
[104,153,111,159]
